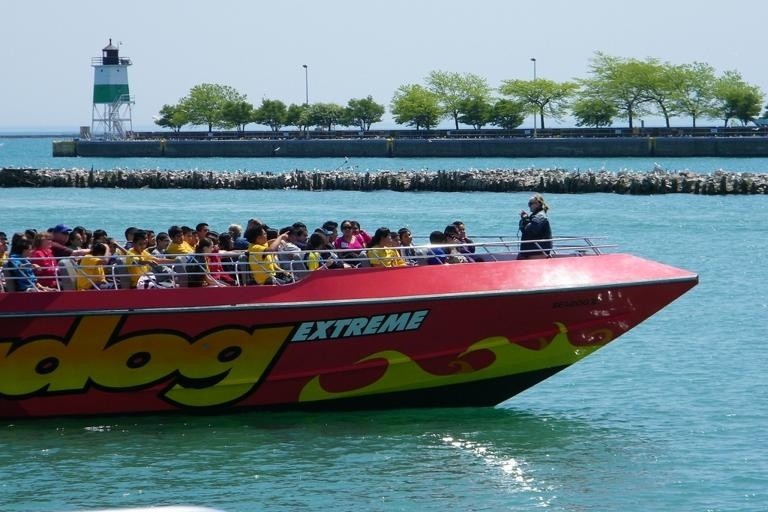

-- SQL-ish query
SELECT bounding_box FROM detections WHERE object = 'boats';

[1,235,701,421]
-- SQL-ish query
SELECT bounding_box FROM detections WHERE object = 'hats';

[55,223,72,233]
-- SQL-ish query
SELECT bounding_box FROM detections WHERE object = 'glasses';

[340,226,353,230]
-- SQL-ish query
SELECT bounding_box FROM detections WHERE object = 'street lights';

[530,58,536,137]
[301,63,310,139]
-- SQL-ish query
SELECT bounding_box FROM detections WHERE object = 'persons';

[1,220,486,292]
[516,194,554,261]
